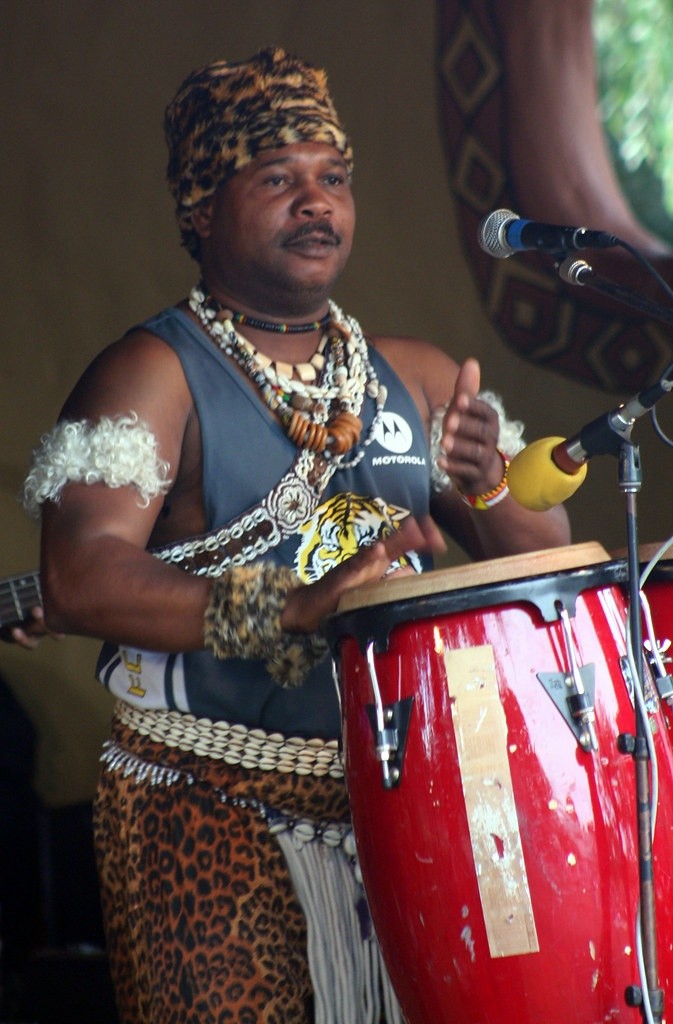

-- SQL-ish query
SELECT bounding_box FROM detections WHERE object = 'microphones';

[477,207,616,258]
[507,381,672,512]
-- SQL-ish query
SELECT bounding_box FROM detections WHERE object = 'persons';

[0,607,67,1024]
[24,42,570,1024]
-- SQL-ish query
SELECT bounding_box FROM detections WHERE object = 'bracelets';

[452,450,510,513]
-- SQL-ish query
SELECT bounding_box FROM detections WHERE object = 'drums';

[330,540,673,1024]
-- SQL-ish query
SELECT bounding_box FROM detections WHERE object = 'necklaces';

[188,277,388,470]
